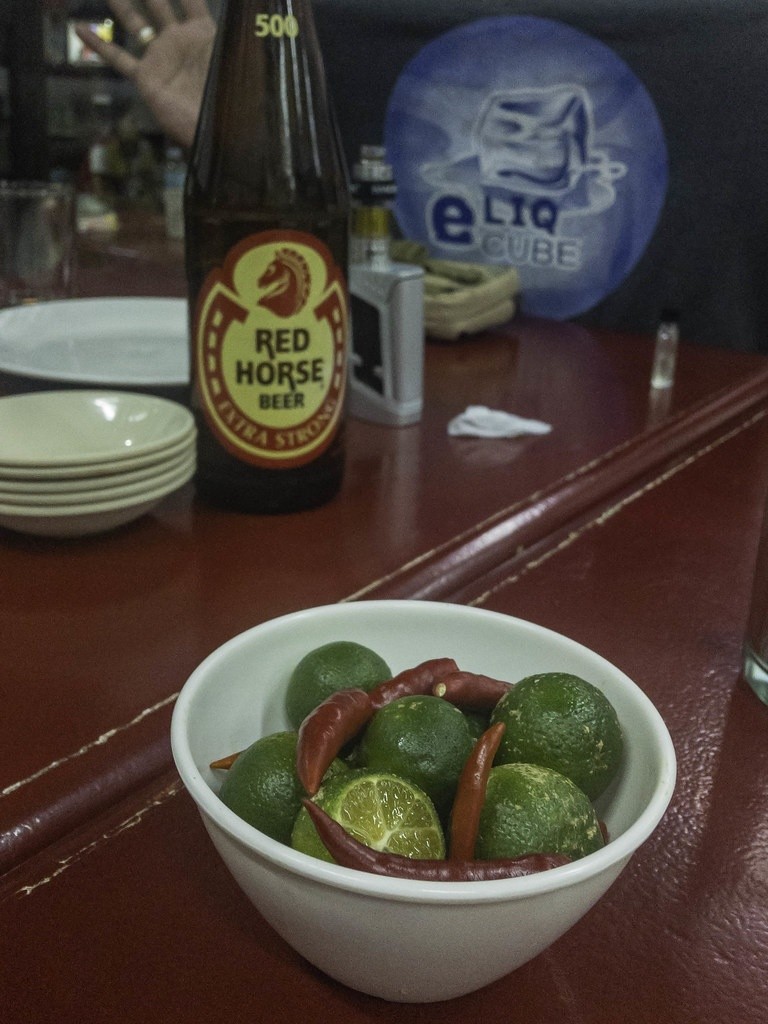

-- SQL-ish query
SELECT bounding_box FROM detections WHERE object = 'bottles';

[344,139,426,428]
[178,0,351,517]
[649,308,679,390]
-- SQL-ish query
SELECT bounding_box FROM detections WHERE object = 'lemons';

[219,640,623,878]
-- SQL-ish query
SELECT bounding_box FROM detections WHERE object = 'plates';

[0,295,191,402]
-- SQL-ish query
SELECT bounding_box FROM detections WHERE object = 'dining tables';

[0,212,768,1024]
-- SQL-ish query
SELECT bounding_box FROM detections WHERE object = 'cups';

[740,500,768,705]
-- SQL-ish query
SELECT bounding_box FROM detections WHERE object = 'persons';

[69,0,457,201]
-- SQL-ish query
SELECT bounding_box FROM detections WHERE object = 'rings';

[134,26,156,48]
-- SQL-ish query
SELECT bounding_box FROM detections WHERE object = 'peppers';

[210,753,238,769]
[433,671,513,712]
[297,687,373,794]
[445,722,505,862]
[367,657,460,711]
[300,796,574,881]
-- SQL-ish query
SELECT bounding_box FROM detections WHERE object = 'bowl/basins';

[0,386,199,539]
[168,594,679,1004]
